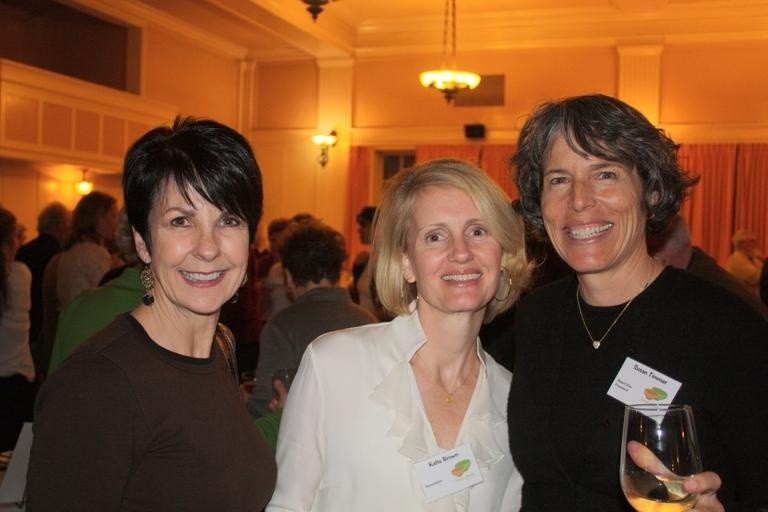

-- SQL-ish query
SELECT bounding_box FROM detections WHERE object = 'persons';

[267,159,538,510]
[480,94,765,512]
[1,188,765,459]
[24,113,278,509]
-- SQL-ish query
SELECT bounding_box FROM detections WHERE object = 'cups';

[619,403,703,512]
[240,367,296,416]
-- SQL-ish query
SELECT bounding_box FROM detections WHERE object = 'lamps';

[76,168,94,196]
[312,130,338,167]
[419,0,481,104]
